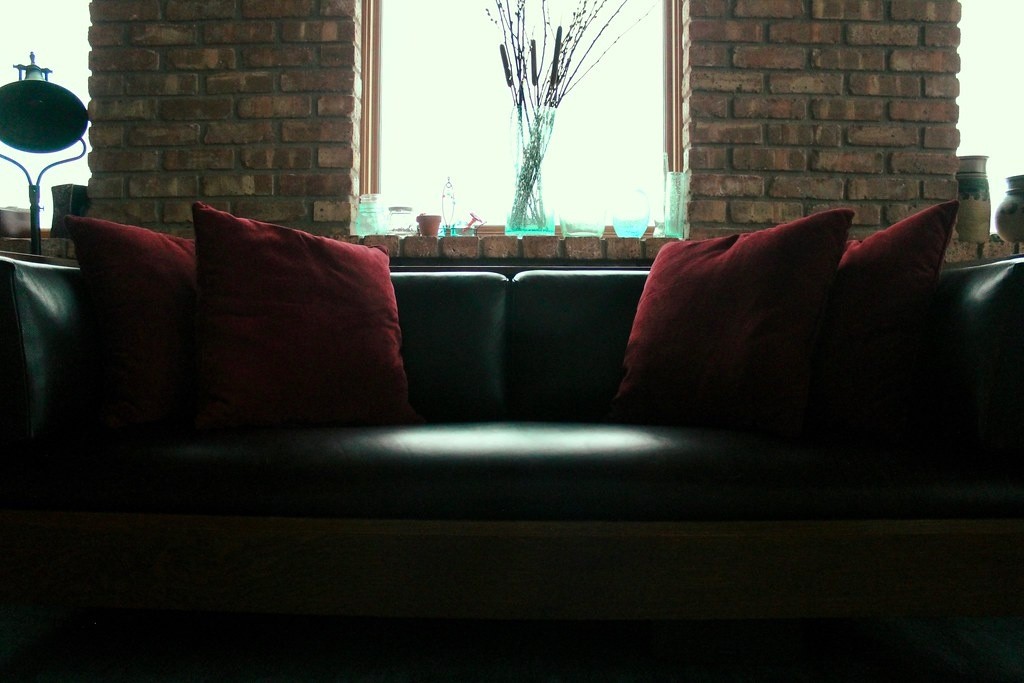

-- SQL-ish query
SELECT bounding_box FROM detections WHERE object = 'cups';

[664,171,687,237]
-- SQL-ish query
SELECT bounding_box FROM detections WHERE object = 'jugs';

[355,194,384,236]
[387,207,414,237]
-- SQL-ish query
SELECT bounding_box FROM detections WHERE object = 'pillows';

[62,212,196,431]
[807,201,960,452]
[598,208,853,439]
[191,199,427,435]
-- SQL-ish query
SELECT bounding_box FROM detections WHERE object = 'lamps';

[0,50,89,255]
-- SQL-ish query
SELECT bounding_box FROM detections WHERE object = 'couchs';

[0,250,1024,647]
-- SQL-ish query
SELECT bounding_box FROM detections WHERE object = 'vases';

[416,215,442,236]
[612,190,651,237]
[958,155,991,242]
[560,203,608,238]
[994,174,1024,243]
[505,104,560,235]
[663,170,688,239]
